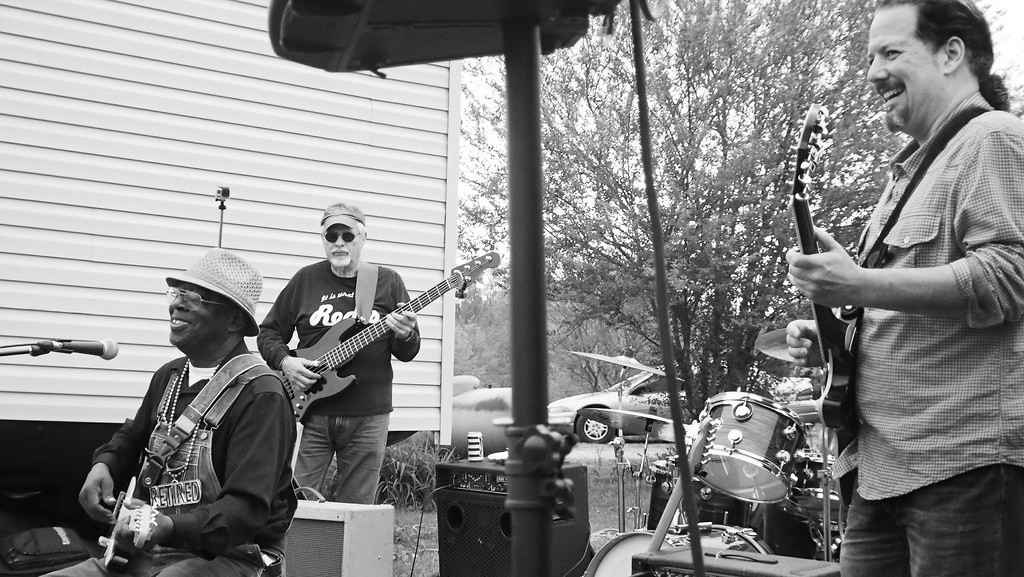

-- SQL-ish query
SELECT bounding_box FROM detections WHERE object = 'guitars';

[783,103,864,507]
[283,252,501,424]
[97,475,158,570]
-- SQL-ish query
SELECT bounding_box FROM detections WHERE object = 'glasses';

[321,232,364,242]
[165,287,238,311]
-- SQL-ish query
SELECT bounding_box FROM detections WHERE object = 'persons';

[784,1,1024,577]
[38,248,300,577]
[257,201,418,505]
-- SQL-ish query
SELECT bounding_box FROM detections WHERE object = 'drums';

[583,523,776,577]
[789,450,840,502]
[749,498,848,563]
[647,459,754,531]
[694,391,807,503]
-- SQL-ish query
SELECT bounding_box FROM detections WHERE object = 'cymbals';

[756,328,797,362]
[567,350,685,382]
[576,407,690,443]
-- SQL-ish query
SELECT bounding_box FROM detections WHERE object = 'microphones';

[37,340,119,360]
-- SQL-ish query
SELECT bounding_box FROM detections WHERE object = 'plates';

[488,452,508,461]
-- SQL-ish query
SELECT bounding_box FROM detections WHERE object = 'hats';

[321,202,366,233]
[166,248,261,337]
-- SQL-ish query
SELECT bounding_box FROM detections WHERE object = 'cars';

[547,361,695,444]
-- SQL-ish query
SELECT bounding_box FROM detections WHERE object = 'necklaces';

[147,358,219,510]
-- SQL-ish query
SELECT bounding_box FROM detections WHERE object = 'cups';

[467,431,484,462]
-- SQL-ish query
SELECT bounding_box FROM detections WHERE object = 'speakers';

[268,0,622,72]
[436,459,591,577]
[283,500,395,577]
